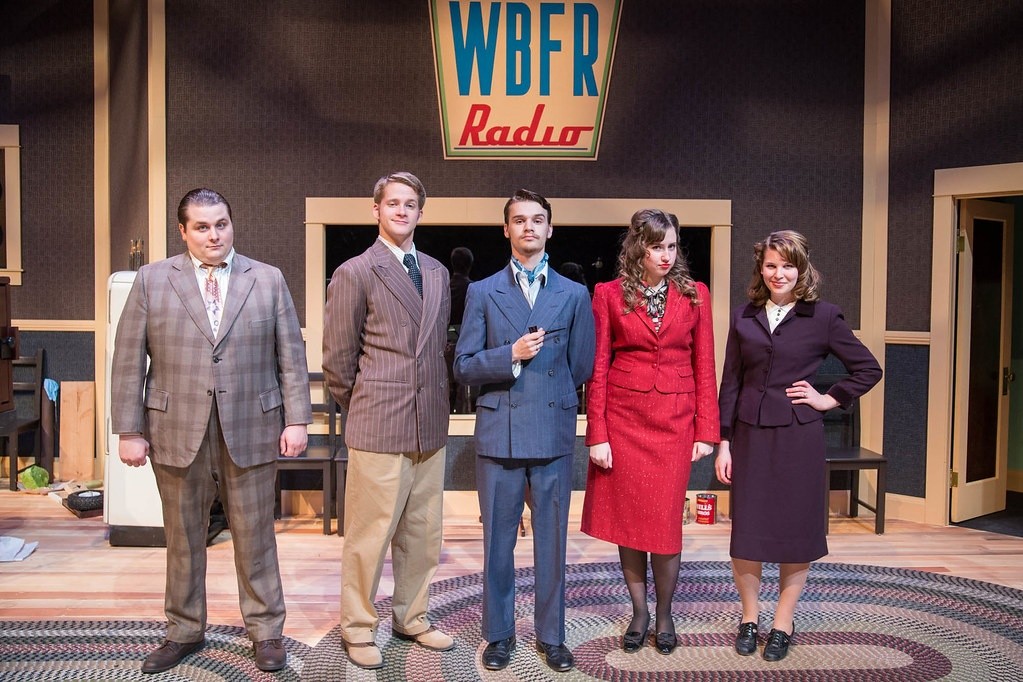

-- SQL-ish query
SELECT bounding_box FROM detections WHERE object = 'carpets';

[0,622,310,681]
[302,560,1022,681]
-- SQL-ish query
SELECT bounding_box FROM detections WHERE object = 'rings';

[805,393,806,397]
[536,345,539,350]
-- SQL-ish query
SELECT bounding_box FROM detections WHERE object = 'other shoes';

[393,625,454,651]
[341,636,384,669]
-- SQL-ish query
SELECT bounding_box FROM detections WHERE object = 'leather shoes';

[141,637,205,673]
[483,636,516,669]
[623,614,650,653]
[655,618,677,655]
[535,637,574,671]
[253,639,286,670]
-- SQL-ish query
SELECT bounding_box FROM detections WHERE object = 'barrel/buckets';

[682,498,691,525]
[696,494,717,525]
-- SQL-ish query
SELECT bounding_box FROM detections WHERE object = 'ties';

[199,262,227,340]
[403,254,423,301]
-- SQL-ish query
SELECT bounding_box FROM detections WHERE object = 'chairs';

[813,373,889,535]
[269,372,339,536]
[0,346,46,492]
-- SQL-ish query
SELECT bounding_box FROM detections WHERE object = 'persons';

[450,248,474,322]
[580,209,719,656]
[454,189,595,671]
[714,229,882,661]
[323,172,455,669]
[112,188,313,672]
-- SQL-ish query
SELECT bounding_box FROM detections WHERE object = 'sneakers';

[763,621,795,662]
[736,614,759,656]
